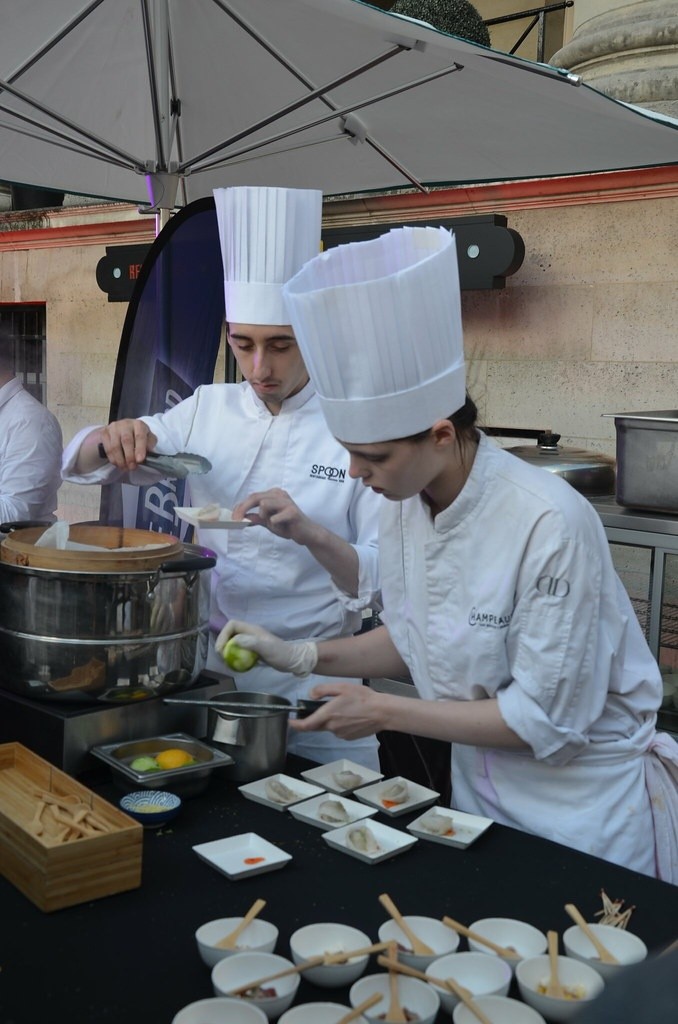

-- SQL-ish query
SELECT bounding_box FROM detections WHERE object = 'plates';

[321,819,418,863]
[173,506,251,529]
[190,833,293,881]
[289,793,377,831]
[406,806,494,849]
[353,775,437,816]
[238,771,326,814]
[301,757,385,796]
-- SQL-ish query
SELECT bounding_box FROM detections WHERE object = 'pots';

[0,519,217,704]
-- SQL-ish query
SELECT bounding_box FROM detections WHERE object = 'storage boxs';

[0,742,143,911]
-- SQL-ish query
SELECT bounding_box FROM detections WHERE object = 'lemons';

[223,635,259,673]
[132,748,194,772]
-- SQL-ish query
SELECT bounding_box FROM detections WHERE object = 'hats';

[281,228,465,443]
[214,186,323,327]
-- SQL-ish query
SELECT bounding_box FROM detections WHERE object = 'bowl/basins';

[171,915,649,1024]
[122,791,182,829]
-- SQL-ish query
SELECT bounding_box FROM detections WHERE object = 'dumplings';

[264,770,453,856]
[198,501,221,522]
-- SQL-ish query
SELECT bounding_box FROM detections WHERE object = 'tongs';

[97,442,213,477]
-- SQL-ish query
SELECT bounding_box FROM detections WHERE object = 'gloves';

[215,620,317,677]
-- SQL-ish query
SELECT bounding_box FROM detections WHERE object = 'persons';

[215,225,678,888]
[0,324,63,526]
[61,185,382,782]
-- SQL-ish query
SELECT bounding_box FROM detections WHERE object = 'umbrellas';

[0,0,678,409]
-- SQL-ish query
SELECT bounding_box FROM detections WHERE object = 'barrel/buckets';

[206,692,288,783]
